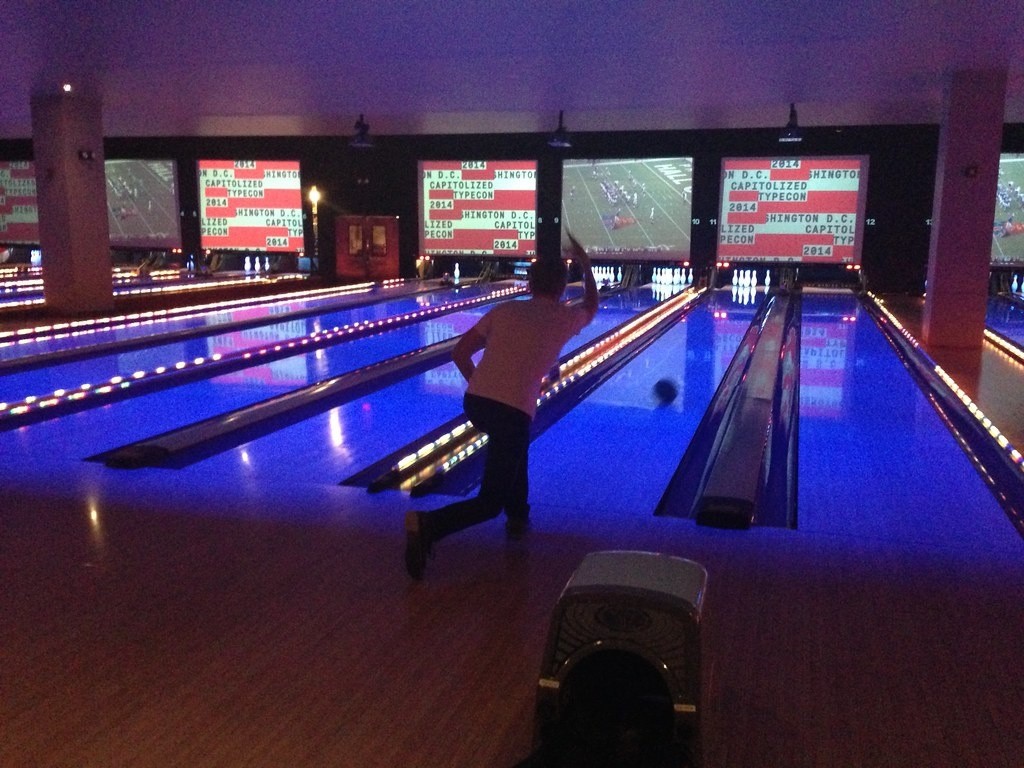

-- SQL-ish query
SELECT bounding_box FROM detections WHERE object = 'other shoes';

[505,518,532,540]
[406,510,435,579]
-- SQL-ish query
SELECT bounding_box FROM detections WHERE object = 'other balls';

[653,378,679,404]
[444,271,450,281]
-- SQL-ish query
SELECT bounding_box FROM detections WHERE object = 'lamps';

[777,103,804,146]
[79,149,97,161]
[349,113,375,150]
[546,109,574,149]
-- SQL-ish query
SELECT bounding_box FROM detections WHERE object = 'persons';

[649,206,655,220]
[570,186,576,201]
[402,232,600,580]
[592,165,646,209]
[998,180,1024,212]
[681,191,687,206]
[1006,213,1014,228]
[111,176,151,214]
[615,208,621,221]
